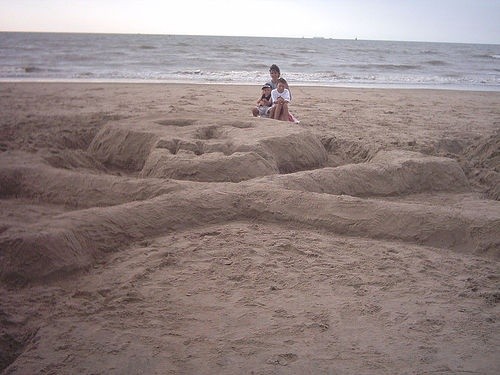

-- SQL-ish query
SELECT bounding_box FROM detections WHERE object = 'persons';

[264,63,300,124]
[253,83,272,117]
[270,78,290,120]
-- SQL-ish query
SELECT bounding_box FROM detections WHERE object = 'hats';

[262,83,272,89]
